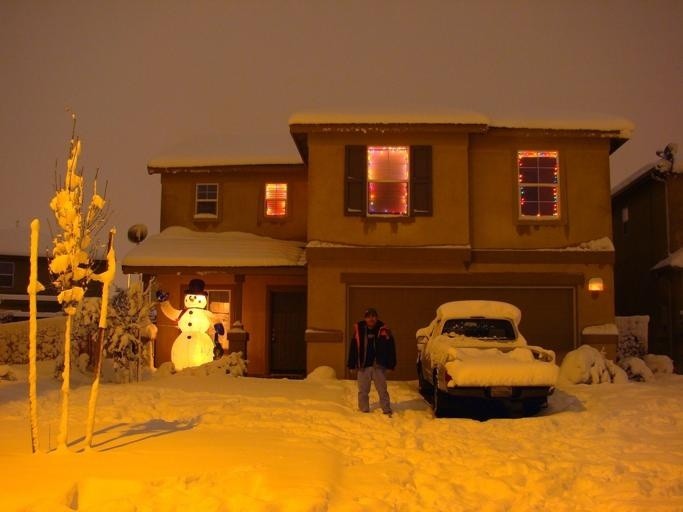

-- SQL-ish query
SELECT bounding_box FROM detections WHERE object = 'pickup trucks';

[410,300,559,418]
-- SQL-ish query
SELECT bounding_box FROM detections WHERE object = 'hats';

[364,307,378,319]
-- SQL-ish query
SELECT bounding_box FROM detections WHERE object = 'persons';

[346,309,396,416]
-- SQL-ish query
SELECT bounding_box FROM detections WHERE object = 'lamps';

[587,275,605,301]
